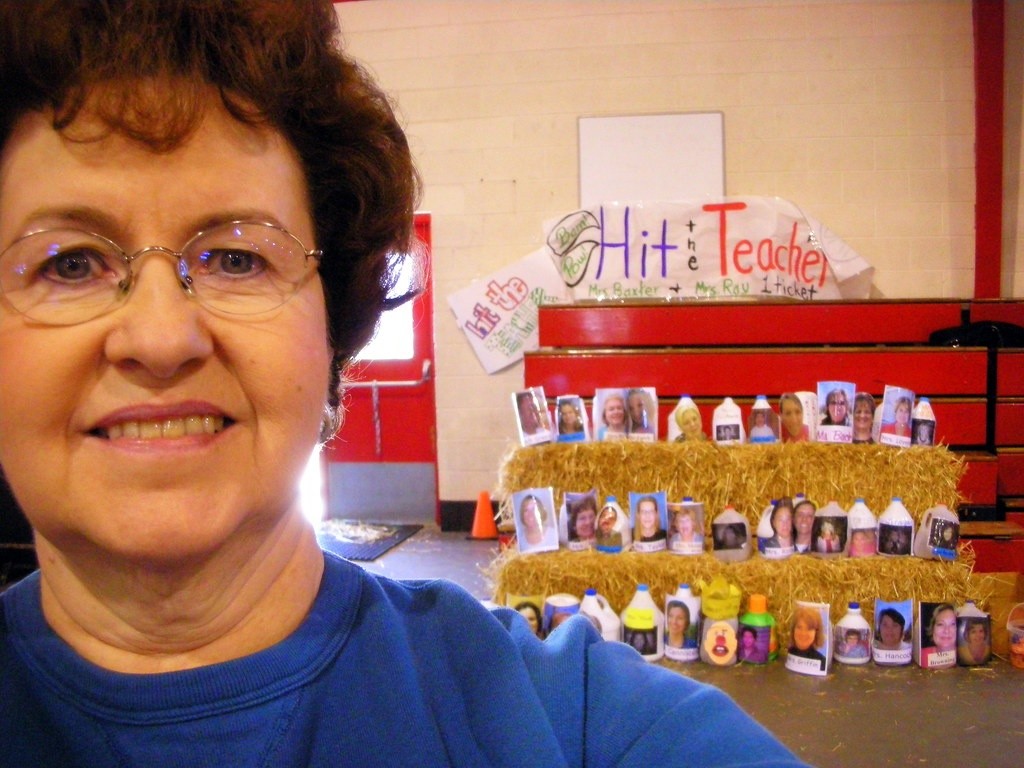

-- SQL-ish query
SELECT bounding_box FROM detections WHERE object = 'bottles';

[666,393,702,443]
[809,499,852,555]
[712,397,747,447]
[592,496,633,554]
[756,496,796,560]
[749,395,782,446]
[736,592,779,664]
[955,600,993,668]
[710,503,753,561]
[791,492,821,556]
[912,501,961,561]
[618,583,703,663]
[477,596,503,613]
[831,601,874,665]
[876,496,915,557]
[845,498,878,558]
[576,588,622,643]
[911,396,936,447]
[665,495,704,555]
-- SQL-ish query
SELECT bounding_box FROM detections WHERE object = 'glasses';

[0,218,324,327]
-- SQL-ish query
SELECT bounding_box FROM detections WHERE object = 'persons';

[0,0,806,768]
[502,380,994,674]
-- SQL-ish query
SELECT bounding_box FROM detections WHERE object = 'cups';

[697,580,746,666]
[542,593,582,640]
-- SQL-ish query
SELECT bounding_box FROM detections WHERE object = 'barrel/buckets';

[1005,601,1024,670]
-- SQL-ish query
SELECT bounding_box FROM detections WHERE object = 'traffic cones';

[464,490,499,541]
